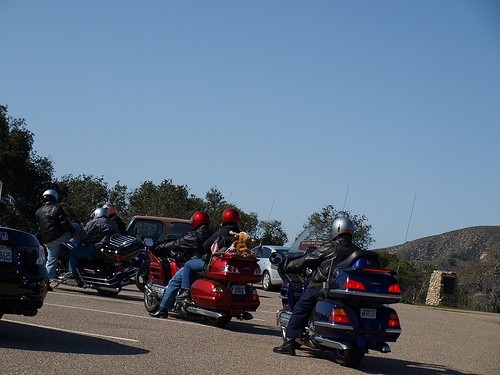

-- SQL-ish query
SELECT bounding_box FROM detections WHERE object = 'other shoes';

[58,271,76,280]
[47,285,53,291]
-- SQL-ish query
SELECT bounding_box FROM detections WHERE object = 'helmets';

[222,209,240,225]
[94,208,107,219]
[332,218,354,239]
[42,189,58,203]
[102,204,116,215]
[191,212,210,230]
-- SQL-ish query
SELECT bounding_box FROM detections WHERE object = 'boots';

[274,338,295,355]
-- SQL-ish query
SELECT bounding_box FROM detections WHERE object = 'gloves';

[155,244,161,249]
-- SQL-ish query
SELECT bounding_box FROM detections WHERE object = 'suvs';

[125,215,195,293]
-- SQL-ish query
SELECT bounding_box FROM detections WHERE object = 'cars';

[251,245,307,292]
[0,225,50,319]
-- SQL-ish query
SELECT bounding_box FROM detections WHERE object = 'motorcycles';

[268,226,403,369]
[34,230,139,297]
[138,237,263,328]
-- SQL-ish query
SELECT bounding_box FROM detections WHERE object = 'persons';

[175,208,246,301]
[35,190,85,292]
[273,216,368,355]
[61,203,128,282]
[148,213,210,319]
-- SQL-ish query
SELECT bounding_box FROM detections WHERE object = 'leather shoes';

[175,292,190,300]
[149,308,168,319]
